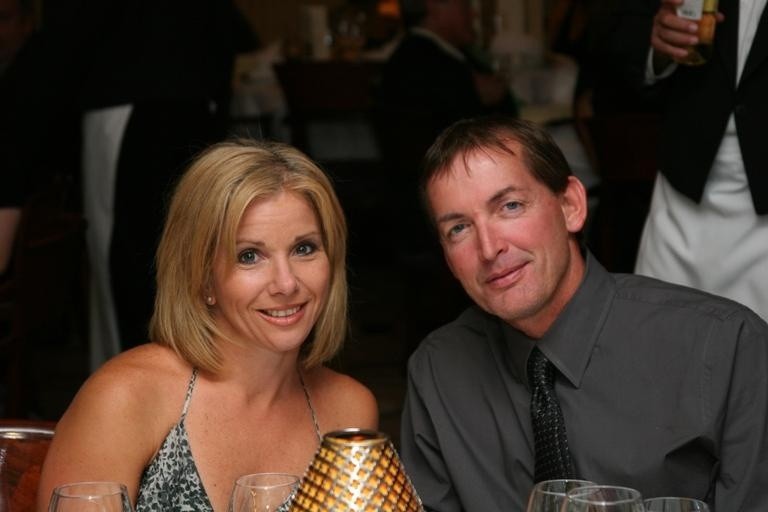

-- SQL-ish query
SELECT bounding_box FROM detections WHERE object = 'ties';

[526,345,577,484]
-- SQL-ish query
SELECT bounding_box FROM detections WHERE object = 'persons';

[398,114,767,511]
[2,2,767,443]
[36,142,380,511]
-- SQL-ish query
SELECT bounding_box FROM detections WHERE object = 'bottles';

[674,0,719,64]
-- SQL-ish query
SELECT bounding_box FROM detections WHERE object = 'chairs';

[0,419,59,511]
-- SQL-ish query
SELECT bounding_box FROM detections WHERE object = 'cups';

[48,481,130,512]
[228,473,300,512]
[528,479,709,512]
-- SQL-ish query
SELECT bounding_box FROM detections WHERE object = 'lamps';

[286,430,427,512]
